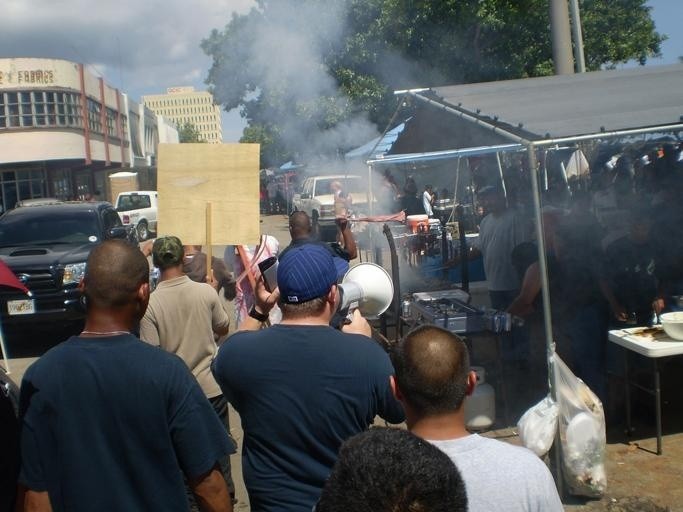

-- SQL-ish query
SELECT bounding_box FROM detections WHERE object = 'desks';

[608,323,682,457]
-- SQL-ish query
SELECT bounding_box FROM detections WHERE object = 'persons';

[0,145,682,511]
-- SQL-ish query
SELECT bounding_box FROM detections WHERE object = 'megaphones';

[337,262,395,323]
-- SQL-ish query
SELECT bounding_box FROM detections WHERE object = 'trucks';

[104,167,156,201]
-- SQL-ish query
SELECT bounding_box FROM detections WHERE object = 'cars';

[14,197,62,208]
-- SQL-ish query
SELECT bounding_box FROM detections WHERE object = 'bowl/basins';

[659,312,682,342]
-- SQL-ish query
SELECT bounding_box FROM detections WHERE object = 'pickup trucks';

[0,200,139,359]
[291,174,377,242]
[113,191,157,242]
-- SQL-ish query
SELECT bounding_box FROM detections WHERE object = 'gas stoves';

[405,297,488,336]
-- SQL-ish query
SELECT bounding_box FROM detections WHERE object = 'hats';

[276,243,348,304]
[477,184,500,195]
[152,236,184,265]
